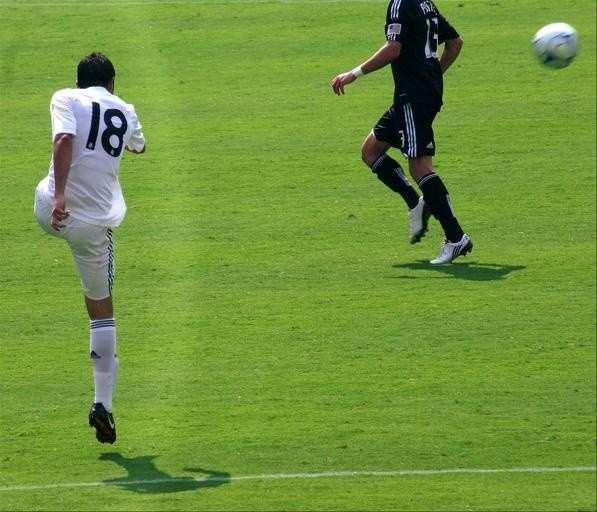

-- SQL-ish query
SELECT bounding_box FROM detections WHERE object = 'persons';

[33,51,148,445]
[330,1,473,265]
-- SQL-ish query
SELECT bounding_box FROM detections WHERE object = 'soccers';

[530,22,579,70]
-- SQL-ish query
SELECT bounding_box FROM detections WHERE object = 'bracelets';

[352,66,362,78]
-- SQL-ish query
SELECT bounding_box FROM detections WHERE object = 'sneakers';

[430,233,474,264]
[88,402,117,444]
[407,195,432,244]
